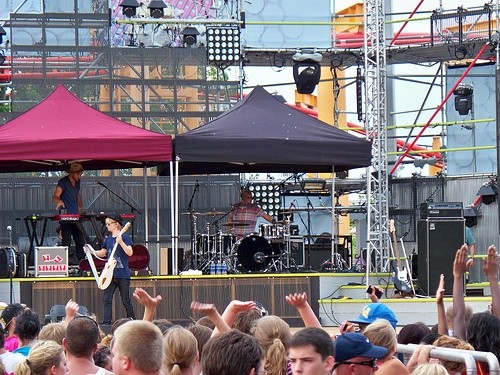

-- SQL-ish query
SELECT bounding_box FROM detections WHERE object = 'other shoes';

[98,321,112,325]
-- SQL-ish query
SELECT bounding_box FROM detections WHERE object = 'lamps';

[206,26,241,63]
[180,27,199,45]
[44,304,66,326]
[147,0,167,18]
[292,53,323,94]
[273,52,286,68]
[0,49,6,65]
[300,179,327,190]
[336,169,349,179]
[118,0,140,17]
[455,46,468,61]
[453,87,473,116]
[330,57,343,68]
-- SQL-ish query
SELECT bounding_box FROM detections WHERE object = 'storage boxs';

[281,236,305,267]
[34,246,69,278]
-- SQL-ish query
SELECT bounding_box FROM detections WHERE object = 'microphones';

[96,181,106,187]
[196,179,199,192]
[319,196,322,205]
[288,200,295,211]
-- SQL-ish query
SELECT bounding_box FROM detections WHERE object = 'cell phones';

[366,285,383,299]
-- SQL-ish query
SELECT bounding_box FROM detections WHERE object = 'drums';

[228,235,273,274]
[193,233,215,256]
[217,234,238,255]
[258,223,286,244]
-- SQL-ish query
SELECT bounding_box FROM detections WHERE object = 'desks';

[302,234,353,272]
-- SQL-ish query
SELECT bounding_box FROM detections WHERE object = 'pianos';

[14,211,56,266]
[55,212,137,250]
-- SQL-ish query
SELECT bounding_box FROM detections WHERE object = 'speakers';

[417,220,465,298]
[305,246,345,270]
[160,248,184,275]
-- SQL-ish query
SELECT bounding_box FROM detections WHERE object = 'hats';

[99,213,124,225]
[0,302,25,329]
[347,303,398,329]
[334,332,390,361]
[66,163,84,174]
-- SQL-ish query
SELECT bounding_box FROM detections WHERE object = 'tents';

[156,84,371,298]
[0,84,175,275]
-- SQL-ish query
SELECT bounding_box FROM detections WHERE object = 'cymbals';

[222,221,250,226]
[203,210,227,216]
[279,209,302,215]
[181,210,203,216]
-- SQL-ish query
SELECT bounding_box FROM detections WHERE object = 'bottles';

[222,261,227,274]
[216,259,222,275]
[210,261,215,275]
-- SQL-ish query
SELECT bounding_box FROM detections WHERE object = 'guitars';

[389,219,412,293]
[98,222,131,290]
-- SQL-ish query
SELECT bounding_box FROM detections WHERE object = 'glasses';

[250,197,254,200]
[106,221,116,226]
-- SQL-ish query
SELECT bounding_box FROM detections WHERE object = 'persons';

[0,244,500,375]
[53,163,86,266]
[83,213,135,325]
[228,191,272,241]
[465,226,476,264]
